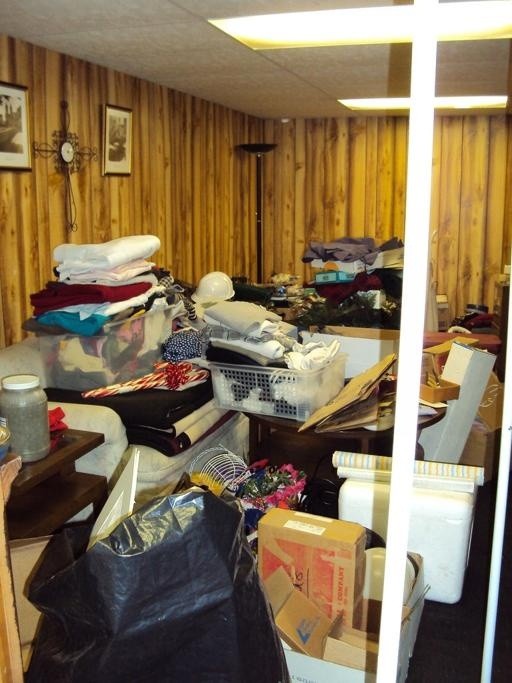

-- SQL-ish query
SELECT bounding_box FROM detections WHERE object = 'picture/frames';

[0,80,34,171]
[101,102,133,177]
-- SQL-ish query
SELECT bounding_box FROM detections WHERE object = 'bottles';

[0,374,50,463]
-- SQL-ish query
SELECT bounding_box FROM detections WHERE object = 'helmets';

[190,271,235,303]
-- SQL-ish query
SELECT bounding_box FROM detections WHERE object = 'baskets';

[208,352,350,423]
[189,443,252,497]
